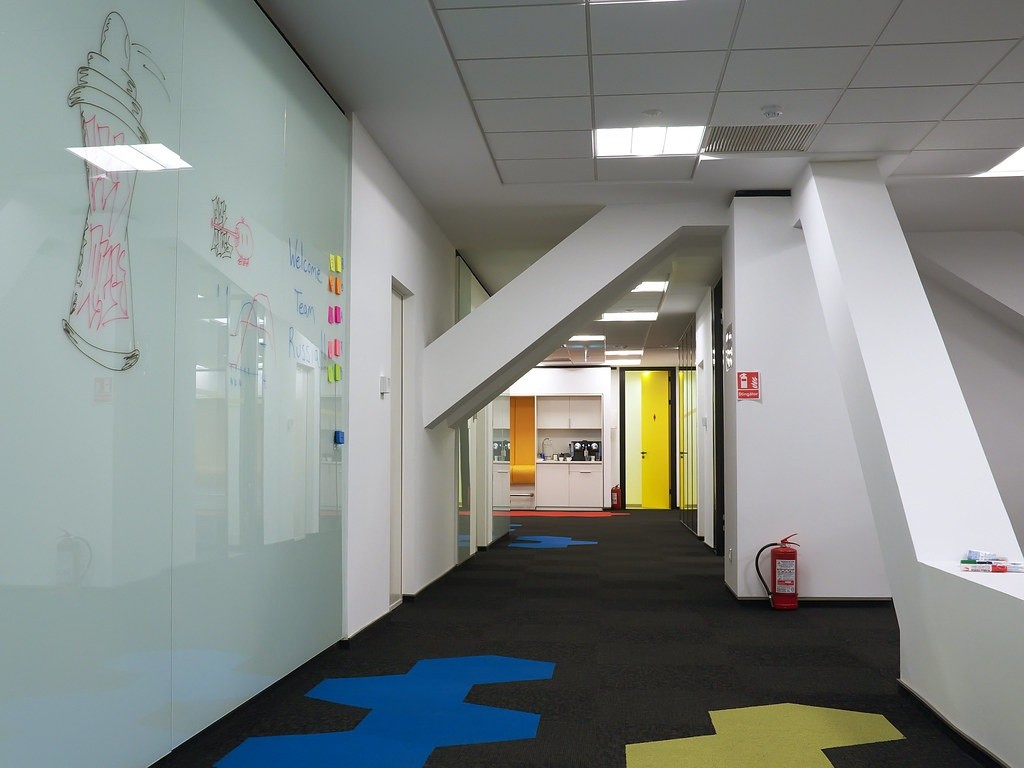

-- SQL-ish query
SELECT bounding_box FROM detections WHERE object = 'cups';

[559,458,564,461]
[566,457,572,462]
[591,456,595,461]
[495,456,499,461]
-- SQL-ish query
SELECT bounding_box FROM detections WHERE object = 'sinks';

[542,458,569,461]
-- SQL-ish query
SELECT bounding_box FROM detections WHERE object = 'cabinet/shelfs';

[534,395,604,511]
[492,461,509,511]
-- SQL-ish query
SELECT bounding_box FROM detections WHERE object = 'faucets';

[541,437,553,453]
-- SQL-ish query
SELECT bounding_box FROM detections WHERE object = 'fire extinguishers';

[754,533,801,612]
[610,484,622,510]
